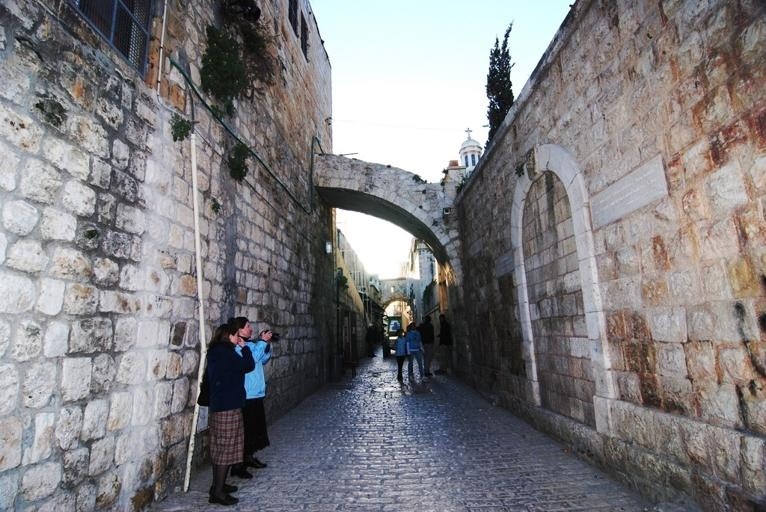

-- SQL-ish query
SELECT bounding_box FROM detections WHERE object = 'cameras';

[260,329,280,342]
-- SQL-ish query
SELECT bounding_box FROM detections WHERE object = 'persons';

[228,316,273,479]
[206,324,256,507]
[392,329,407,387]
[416,315,435,377]
[365,321,380,358]
[435,314,453,375]
[405,322,428,383]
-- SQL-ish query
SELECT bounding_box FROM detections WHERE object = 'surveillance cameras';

[443,207,451,215]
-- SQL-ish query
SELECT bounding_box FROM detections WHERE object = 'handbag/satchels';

[197,368,210,406]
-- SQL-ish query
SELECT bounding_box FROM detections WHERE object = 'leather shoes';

[208,457,267,505]
